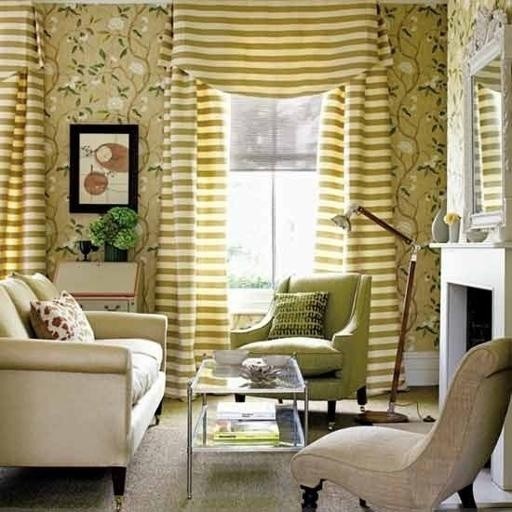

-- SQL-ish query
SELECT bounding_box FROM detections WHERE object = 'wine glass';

[80,241,91,262]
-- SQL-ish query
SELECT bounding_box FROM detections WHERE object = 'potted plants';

[89,205,139,261]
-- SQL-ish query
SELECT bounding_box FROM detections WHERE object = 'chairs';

[237,272,372,427]
[289,337,511,511]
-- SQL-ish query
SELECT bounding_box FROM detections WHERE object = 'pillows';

[29,291,95,345]
[267,292,330,340]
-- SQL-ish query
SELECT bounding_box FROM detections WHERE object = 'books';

[214,420,279,440]
[216,401,276,421]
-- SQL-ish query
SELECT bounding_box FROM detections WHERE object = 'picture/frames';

[69,124,140,214]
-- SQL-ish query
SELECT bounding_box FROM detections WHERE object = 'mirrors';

[459,7,512,234]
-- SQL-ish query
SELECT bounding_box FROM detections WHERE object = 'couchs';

[1,272,169,496]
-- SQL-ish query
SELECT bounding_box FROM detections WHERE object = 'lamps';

[329,202,421,425]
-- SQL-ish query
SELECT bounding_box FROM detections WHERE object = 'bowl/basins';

[466,231,487,243]
[213,350,250,364]
